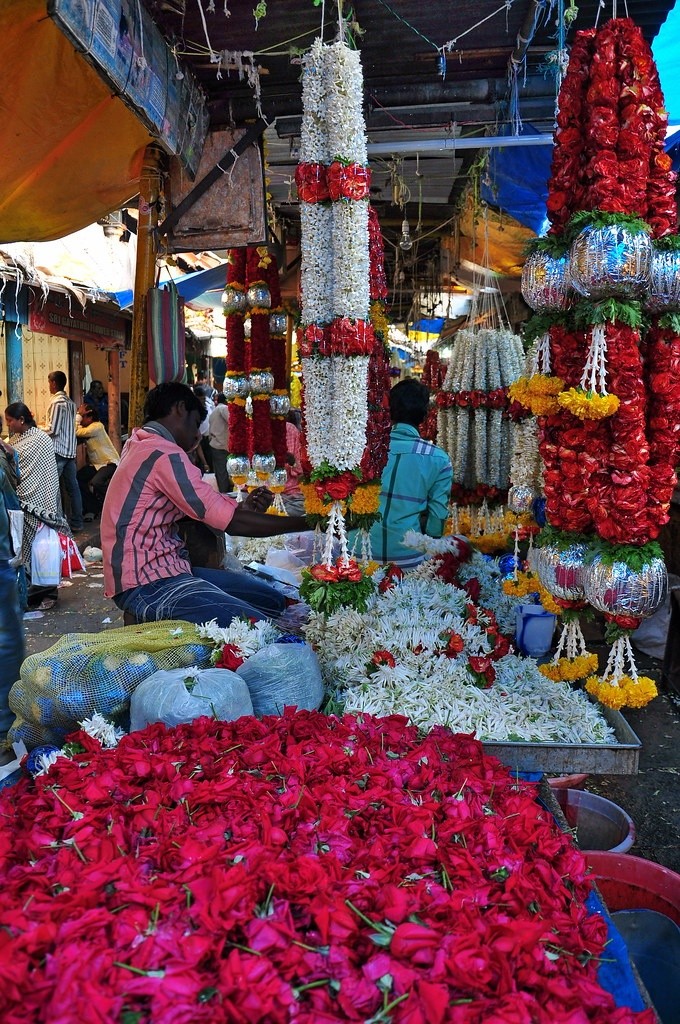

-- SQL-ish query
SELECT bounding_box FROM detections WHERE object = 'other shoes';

[38,598,56,610]
[84,512,95,521]
[69,525,84,533]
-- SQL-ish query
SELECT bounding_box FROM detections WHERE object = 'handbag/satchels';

[238,644,325,718]
[129,667,252,736]
[31,523,63,587]
[57,531,87,579]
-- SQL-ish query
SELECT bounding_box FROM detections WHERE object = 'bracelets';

[76,412,83,417]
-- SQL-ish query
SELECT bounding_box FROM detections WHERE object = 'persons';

[47,370,122,532]
[0,416,27,768]
[187,369,233,494]
[4,403,72,617]
[100,382,357,630]
[344,379,471,579]
[285,420,303,477]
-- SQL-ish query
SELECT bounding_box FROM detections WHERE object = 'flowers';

[0,18,680,1024]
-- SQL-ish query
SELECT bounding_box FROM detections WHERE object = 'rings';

[80,405,82,406]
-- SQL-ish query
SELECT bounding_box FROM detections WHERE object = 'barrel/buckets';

[581,849,680,1024]
[550,787,636,852]
[515,604,556,657]
